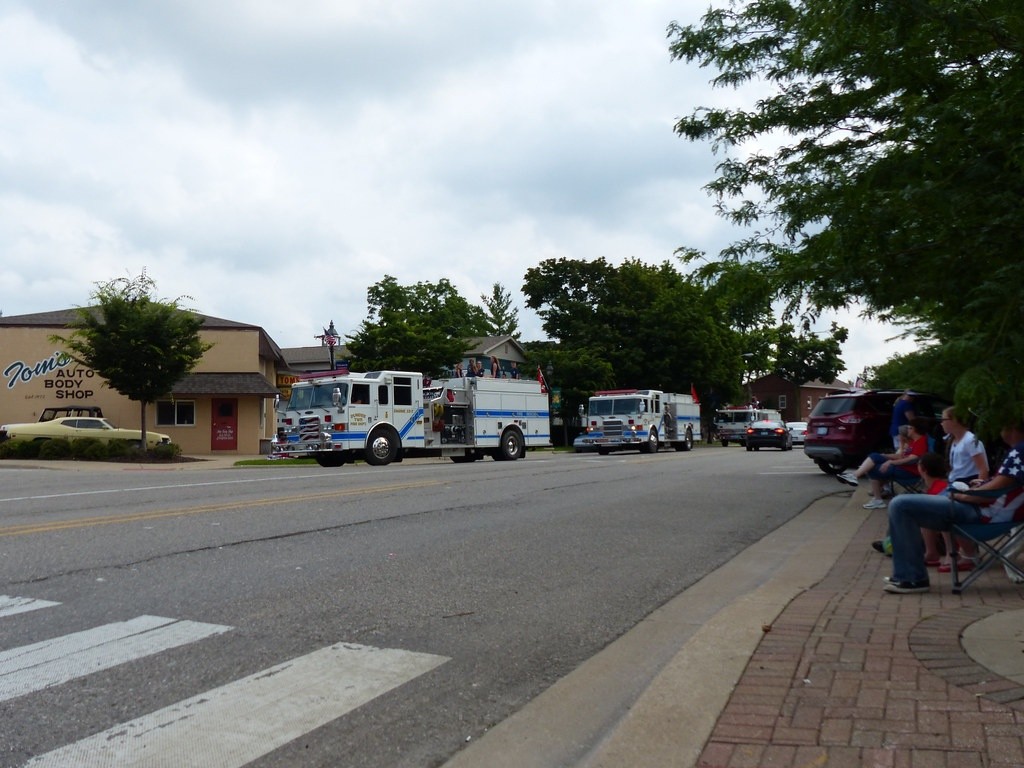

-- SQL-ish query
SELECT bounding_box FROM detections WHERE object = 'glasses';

[939,418,952,423]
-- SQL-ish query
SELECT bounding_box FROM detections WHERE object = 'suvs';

[805,387,940,473]
[0,405,103,440]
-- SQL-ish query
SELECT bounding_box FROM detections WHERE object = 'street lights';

[325,320,339,370]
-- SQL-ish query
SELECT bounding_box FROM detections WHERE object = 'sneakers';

[836,473,859,486]
[863,498,887,509]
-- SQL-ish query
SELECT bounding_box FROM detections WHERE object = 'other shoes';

[882,575,901,585]
[868,489,890,499]
[873,540,893,557]
[884,581,929,594]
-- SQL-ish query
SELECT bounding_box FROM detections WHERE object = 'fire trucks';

[268,369,555,466]
[579,389,701,454]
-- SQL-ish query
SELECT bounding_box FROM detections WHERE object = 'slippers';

[924,560,940,566]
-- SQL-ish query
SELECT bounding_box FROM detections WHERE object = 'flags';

[538,368,545,392]
[691,382,700,404]
[323,327,336,346]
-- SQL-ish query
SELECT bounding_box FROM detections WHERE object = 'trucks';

[714,407,781,447]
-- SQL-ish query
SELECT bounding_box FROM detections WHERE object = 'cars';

[5,416,172,451]
[745,419,793,450]
[785,422,809,443]
[572,431,596,453]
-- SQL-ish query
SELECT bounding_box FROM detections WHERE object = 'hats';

[904,388,917,395]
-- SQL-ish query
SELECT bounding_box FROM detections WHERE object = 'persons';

[837,388,1024,594]
[455,356,520,380]
[351,389,362,404]
[751,396,762,410]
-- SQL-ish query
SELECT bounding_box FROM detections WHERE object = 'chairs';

[890,453,933,497]
[943,476,1024,595]
